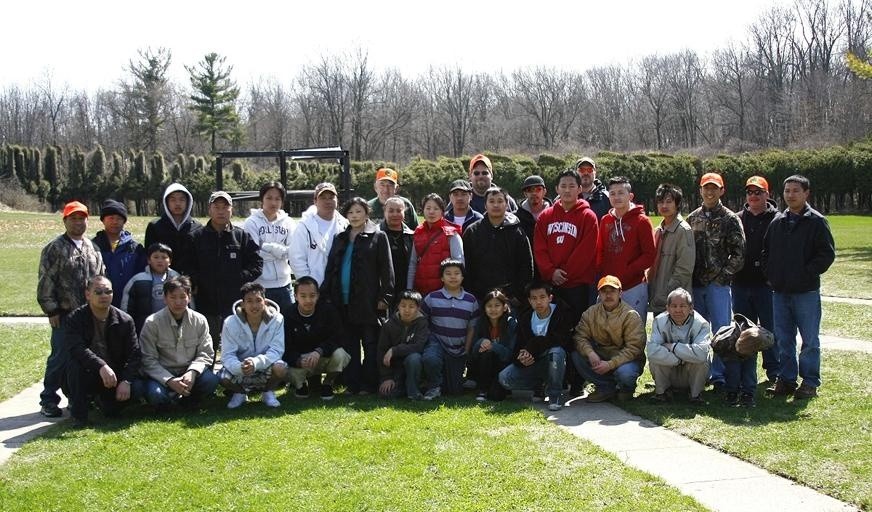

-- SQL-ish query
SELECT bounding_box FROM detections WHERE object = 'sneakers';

[42,402,62,418]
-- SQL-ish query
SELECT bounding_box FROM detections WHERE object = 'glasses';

[474,170,489,176]
[746,189,762,196]
[600,288,618,297]
[425,205,441,213]
[93,289,113,295]
[526,186,542,193]
[577,167,594,173]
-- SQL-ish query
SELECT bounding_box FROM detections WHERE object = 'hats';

[209,191,233,206]
[376,168,397,186]
[521,176,545,190]
[314,182,338,199]
[700,173,724,188]
[746,176,769,194]
[449,180,472,193]
[100,199,127,223]
[63,201,88,219]
[576,157,595,170]
[470,154,492,174]
[597,275,622,291]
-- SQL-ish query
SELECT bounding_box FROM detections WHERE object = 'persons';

[39,153,835,418]
